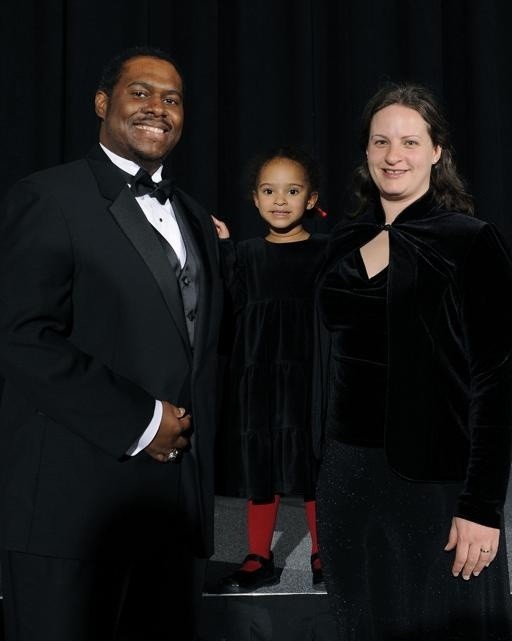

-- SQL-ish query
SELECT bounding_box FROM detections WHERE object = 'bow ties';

[125,167,177,207]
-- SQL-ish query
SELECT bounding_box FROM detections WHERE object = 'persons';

[2,46,224,641]
[309,78,512,641]
[212,143,331,594]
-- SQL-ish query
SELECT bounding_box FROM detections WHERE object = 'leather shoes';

[216,548,276,591]
[308,548,328,591]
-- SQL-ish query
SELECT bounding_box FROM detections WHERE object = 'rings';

[479,549,490,554]
[167,449,179,463]
